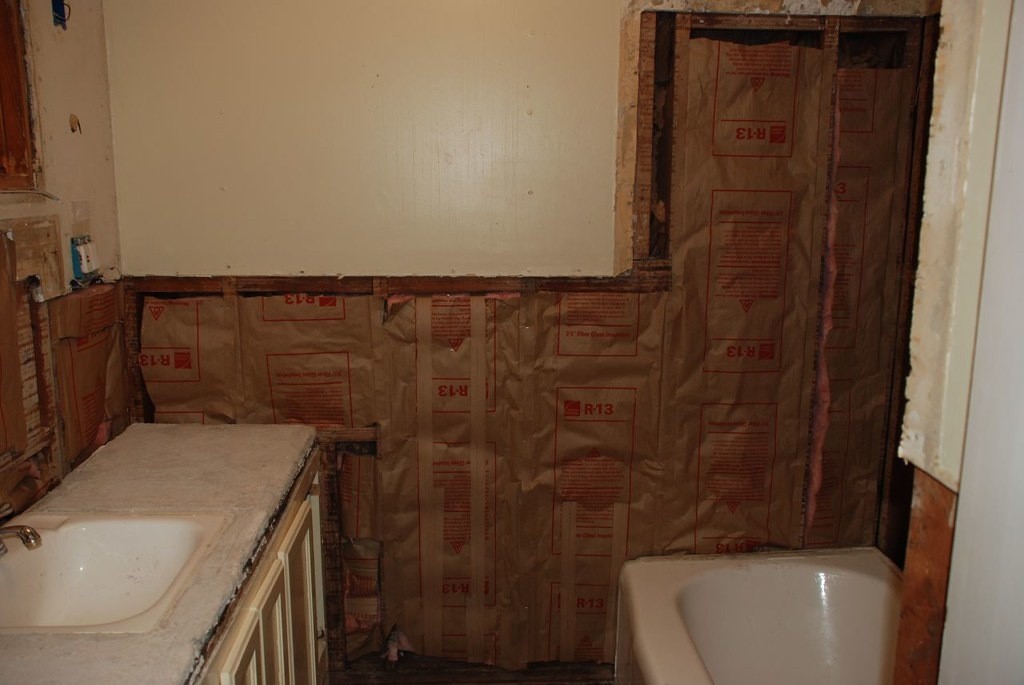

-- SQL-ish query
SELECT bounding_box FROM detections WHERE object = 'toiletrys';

[71,234,100,284]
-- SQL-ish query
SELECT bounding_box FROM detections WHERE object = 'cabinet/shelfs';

[193,443,330,685]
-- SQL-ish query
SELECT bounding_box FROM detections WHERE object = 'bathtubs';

[616,541,902,682]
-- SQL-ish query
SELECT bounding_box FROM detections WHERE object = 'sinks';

[0,507,236,636]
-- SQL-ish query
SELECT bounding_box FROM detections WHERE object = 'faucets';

[0,504,42,555]
